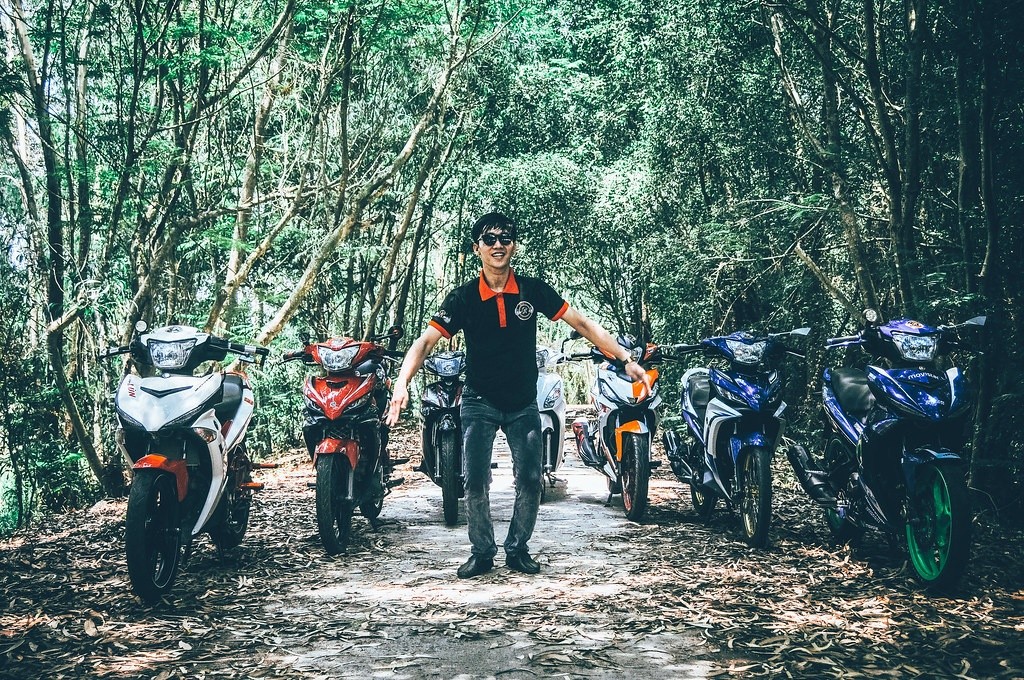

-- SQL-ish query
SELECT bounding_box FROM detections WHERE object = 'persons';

[383,213,654,573]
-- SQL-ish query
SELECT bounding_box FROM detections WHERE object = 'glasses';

[476,232,514,246]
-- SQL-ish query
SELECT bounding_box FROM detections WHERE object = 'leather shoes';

[506,550,541,574]
[457,555,494,579]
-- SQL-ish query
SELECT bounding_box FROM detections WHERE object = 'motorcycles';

[660,325,812,549]
[418,349,498,526]
[784,307,988,600]
[98,319,278,598]
[535,328,681,521]
[277,325,411,555]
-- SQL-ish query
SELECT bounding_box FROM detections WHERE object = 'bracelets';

[623,357,636,365]
